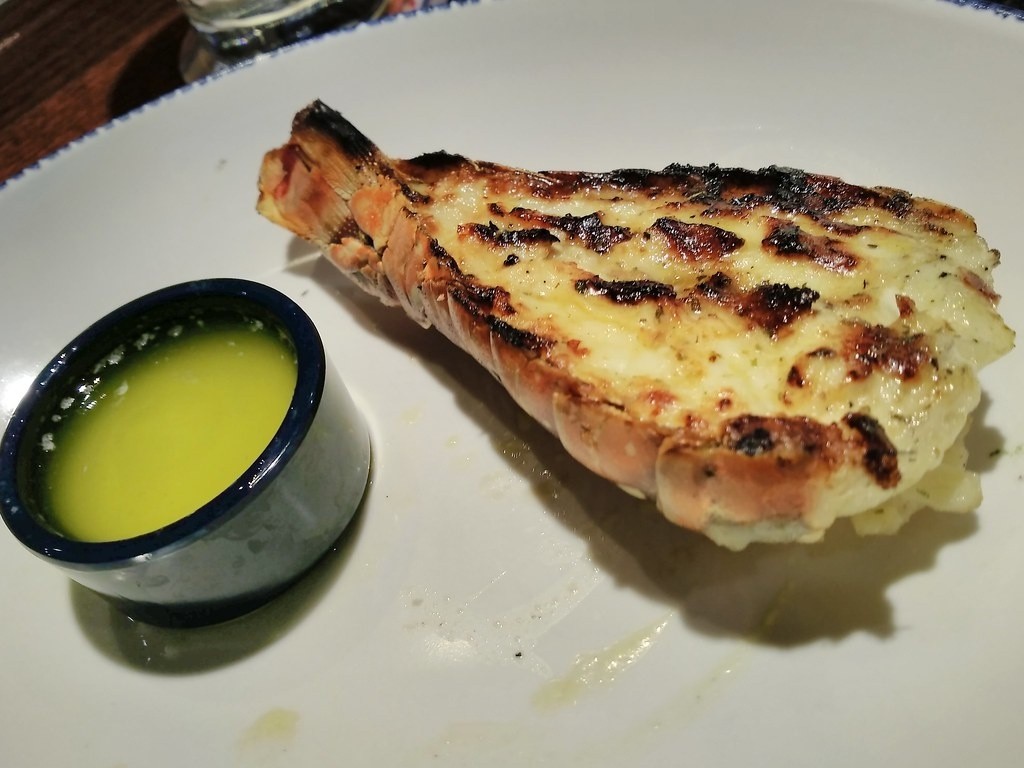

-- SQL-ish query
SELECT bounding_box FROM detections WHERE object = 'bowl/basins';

[0,281,374,632]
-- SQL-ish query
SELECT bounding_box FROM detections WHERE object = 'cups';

[182,0,349,62]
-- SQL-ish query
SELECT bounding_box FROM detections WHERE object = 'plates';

[0,0,1024,768]
